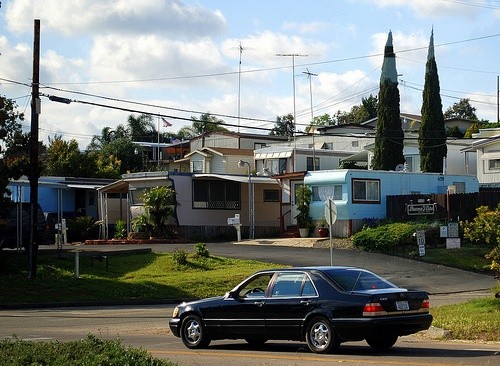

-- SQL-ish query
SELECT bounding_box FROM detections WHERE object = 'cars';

[169,265,434,353]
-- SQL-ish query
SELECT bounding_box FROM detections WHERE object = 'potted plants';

[298,219,310,238]
[316,218,329,237]
[131,214,148,232]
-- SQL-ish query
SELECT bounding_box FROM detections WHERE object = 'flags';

[162,117,173,127]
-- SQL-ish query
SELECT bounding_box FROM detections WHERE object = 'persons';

[55,219,63,246]
[61,219,69,246]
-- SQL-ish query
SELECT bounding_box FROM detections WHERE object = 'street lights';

[238,160,253,240]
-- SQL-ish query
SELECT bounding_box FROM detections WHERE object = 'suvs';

[1,202,45,251]
[41,211,78,245]
